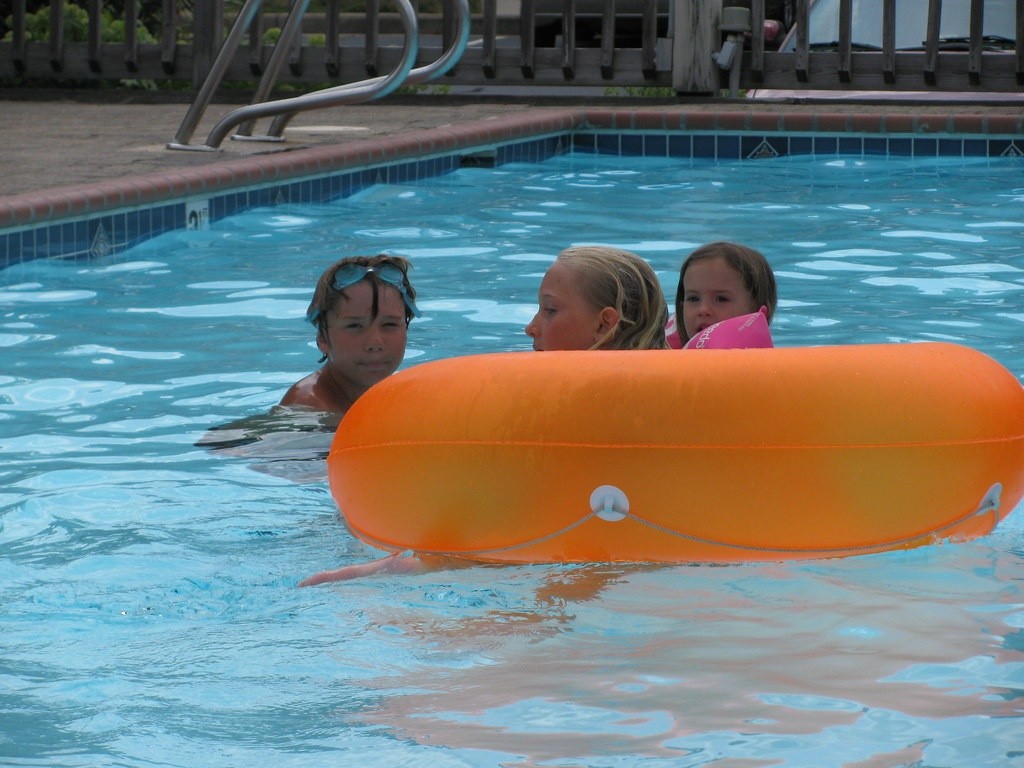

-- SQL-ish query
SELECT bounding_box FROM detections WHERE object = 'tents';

[777,0,1020,53]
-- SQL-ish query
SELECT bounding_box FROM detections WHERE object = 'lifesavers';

[326,343,1024,563]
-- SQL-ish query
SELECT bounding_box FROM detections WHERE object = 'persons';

[676,242,777,345]
[193,252,416,482]
[298,243,671,601]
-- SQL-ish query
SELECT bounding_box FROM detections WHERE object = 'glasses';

[309,263,423,325]
